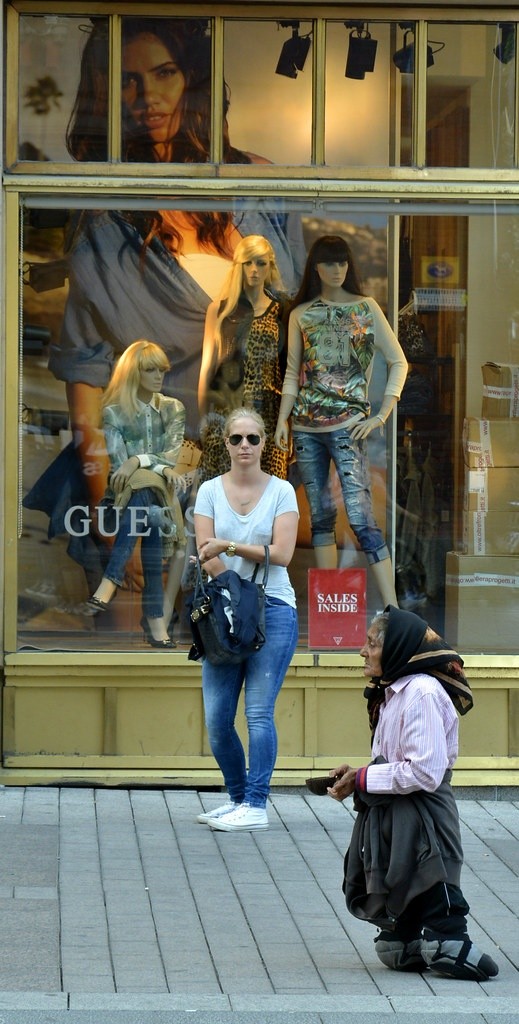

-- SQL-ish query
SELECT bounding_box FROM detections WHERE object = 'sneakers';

[207,803,270,831]
[198,800,241,823]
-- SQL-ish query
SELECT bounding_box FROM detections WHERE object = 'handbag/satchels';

[191,545,269,667]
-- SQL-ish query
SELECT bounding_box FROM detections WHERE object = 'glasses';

[228,433,262,446]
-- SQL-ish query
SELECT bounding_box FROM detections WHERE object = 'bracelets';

[226,540,237,557]
[376,413,385,425]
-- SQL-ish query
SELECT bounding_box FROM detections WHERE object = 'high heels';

[140,616,177,648]
[86,588,117,611]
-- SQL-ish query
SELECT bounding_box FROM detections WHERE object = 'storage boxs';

[463,417,519,468]
[462,510,519,556]
[463,464,519,511]
[480,362,519,417]
[446,552,519,652]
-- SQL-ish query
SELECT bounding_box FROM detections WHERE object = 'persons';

[82,232,408,649]
[20,13,410,636]
[325,604,499,983]
[194,404,300,834]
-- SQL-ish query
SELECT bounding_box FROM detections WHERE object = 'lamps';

[492,24,516,64]
[345,23,378,81]
[276,21,311,78]
[393,22,445,73]
